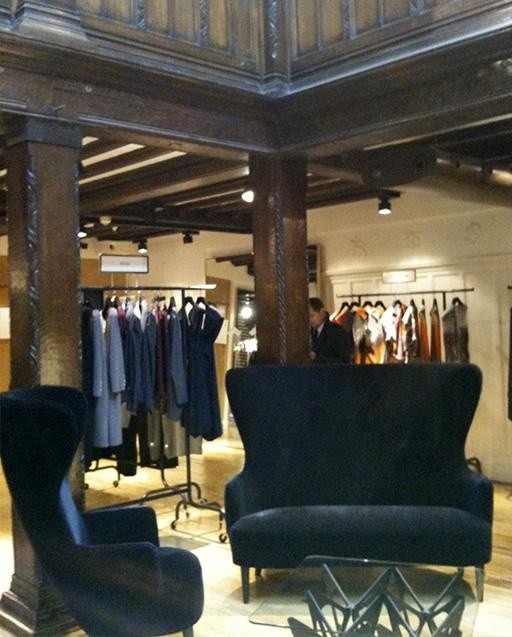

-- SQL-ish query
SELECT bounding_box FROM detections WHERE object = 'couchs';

[223,359,496,602]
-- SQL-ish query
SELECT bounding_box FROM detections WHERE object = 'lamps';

[133,238,148,253]
[378,198,391,215]
[182,229,200,244]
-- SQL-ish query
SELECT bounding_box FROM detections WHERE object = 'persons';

[308,298,349,363]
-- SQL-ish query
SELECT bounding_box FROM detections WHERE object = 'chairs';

[1,382,204,636]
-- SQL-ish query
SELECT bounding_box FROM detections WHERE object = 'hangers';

[335,287,462,323]
[102,282,210,317]
